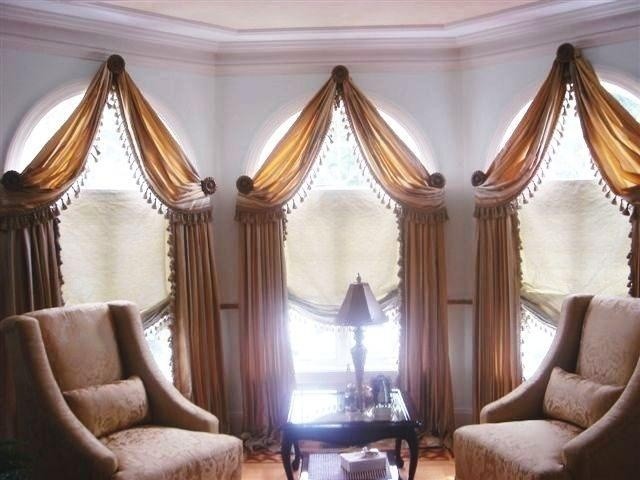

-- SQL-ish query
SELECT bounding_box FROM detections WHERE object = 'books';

[340,451,386,473]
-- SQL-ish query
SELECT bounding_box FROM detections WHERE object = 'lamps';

[335,273,389,401]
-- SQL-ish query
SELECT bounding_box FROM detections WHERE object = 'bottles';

[373,376,390,404]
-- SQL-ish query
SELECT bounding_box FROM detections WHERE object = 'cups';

[337,385,345,413]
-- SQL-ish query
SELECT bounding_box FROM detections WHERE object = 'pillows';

[63,374,153,440]
[541,366,626,430]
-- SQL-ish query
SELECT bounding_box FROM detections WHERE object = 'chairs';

[0,300,243,480]
[453,293,640,480]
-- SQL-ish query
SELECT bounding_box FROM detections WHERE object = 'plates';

[365,452,378,458]
